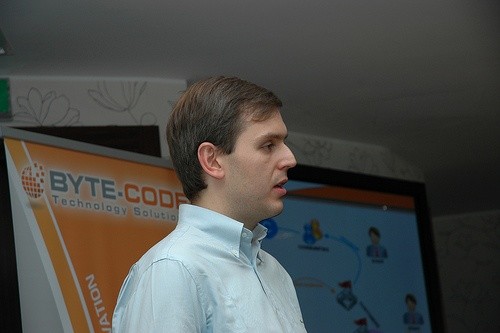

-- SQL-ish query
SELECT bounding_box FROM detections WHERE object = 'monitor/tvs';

[258,163,444,333]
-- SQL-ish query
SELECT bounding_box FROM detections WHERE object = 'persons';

[111,75,306,333]
